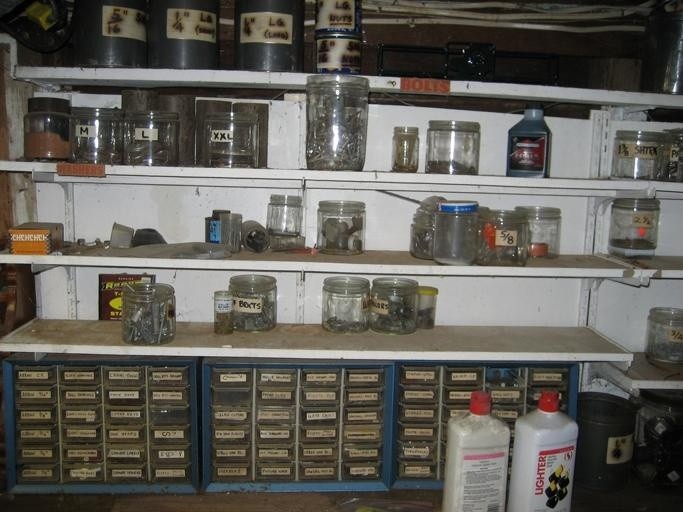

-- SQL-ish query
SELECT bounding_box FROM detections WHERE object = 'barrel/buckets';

[577,391,636,490]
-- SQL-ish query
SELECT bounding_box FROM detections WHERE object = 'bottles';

[504,108,552,180]
[507,391,581,512]
[612,127,683,249]
[407,197,563,266]
[204,192,308,254]
[644,306,683,370]
[121,284,175,346]
[213,291,234,336]
[315,200,366,255]
[390,125,422,173]
[369,279,417,334]
[21,77,270,179]
[320,277,369,333]
[441,390,512,512]
[417,286,437,329]
[301,74,371,172]
[228,275,277,333]
[424,119,482,176]
